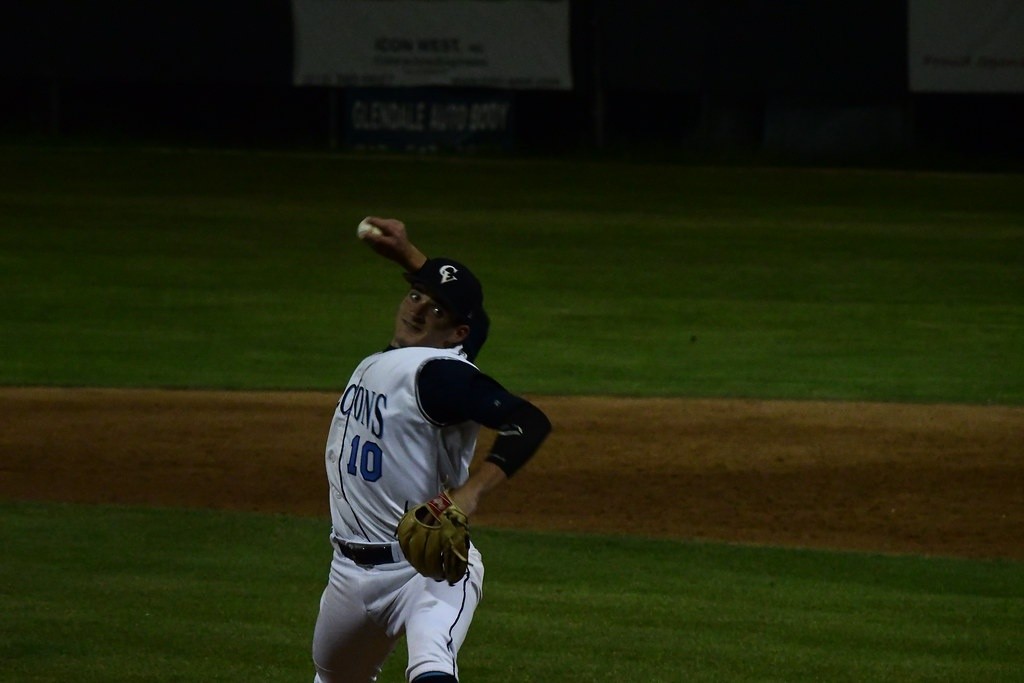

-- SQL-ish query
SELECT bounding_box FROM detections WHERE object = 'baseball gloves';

[397,492,471,583]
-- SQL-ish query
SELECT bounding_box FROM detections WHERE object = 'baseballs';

[358,218,383,239]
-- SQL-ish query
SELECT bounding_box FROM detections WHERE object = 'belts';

[336,540,396,565]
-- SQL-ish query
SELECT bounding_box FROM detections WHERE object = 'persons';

[309,217,551,683]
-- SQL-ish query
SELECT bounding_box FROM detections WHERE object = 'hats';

[402,257,481,323]
